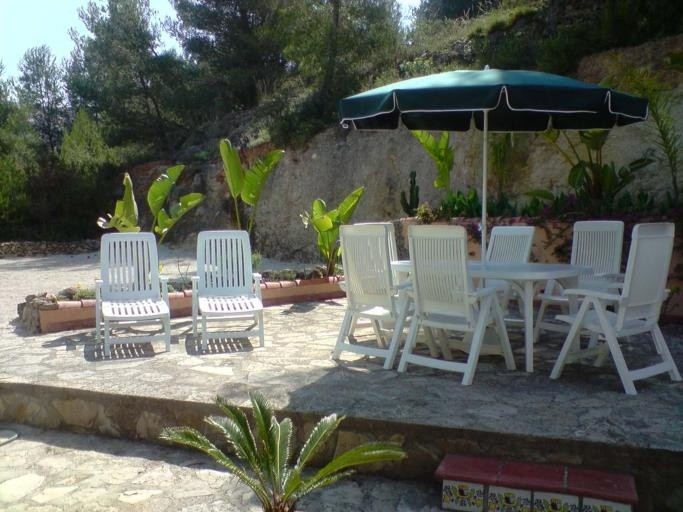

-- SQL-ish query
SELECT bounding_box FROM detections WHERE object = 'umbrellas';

[336,63,649,264]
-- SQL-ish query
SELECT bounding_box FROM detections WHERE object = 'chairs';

[94,226,266,358]
